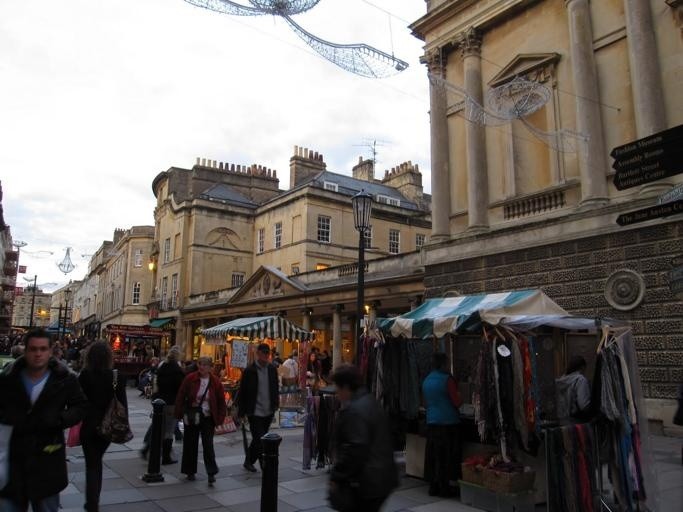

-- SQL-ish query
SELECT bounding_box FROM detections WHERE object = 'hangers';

[597,322,633,355]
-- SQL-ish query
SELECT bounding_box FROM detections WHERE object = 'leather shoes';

[162,458,178,464]
[186,473,196,480]
[207,473,215,484]
[140,448,148,461]
[243,461,257,472]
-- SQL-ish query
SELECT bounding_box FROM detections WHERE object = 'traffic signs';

[608,124,683,192]
[615,199,683,227]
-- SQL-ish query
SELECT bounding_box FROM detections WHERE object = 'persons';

[326,364,400,512]
[556,354,590,415]
[421,350,465,499]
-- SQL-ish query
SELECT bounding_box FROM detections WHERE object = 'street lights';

[350,186,374,365]
[61,285,73,339]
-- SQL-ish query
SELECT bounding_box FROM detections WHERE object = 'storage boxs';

[458,481,538,512]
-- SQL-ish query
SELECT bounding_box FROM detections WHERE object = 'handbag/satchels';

[67,422,82,448]
[183,408,202,426]
[102,397,133,443]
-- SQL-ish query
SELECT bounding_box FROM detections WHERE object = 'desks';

[404,411,556,505]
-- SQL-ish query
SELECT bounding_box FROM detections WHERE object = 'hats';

[258,344,270,351]
